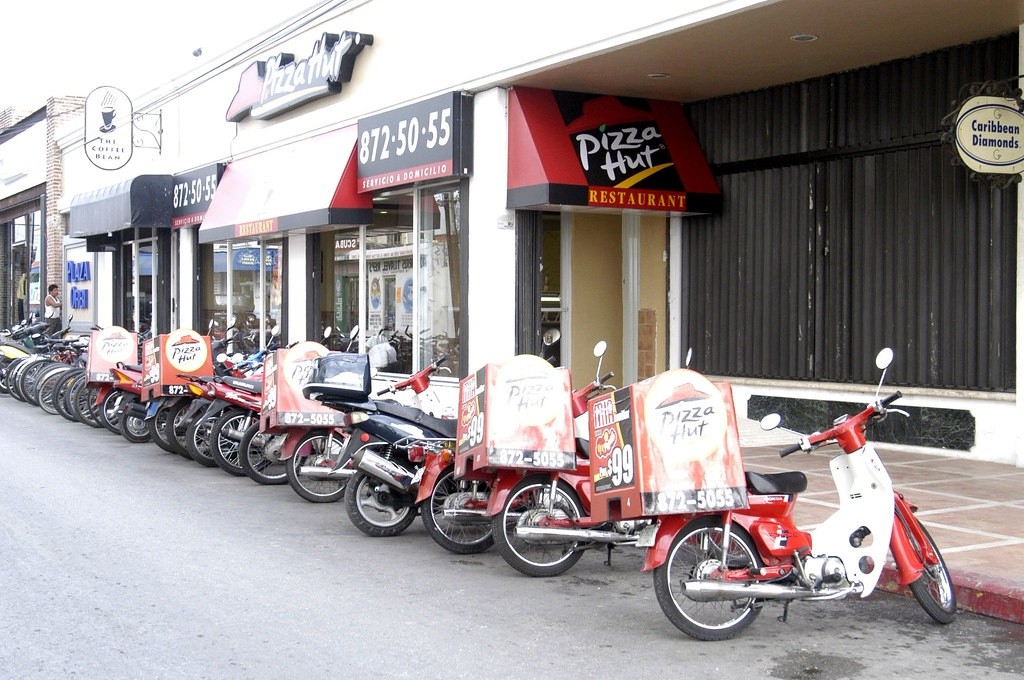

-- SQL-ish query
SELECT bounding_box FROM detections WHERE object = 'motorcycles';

[475,338,652,577]
[641,345,964,637]
[0,314,500,552]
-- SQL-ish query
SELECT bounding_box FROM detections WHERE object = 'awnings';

[69,174,174,238]
[198,138,373,243]
[507,86,722,217]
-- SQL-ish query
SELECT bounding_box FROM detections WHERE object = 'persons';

[44,285,62,337]
